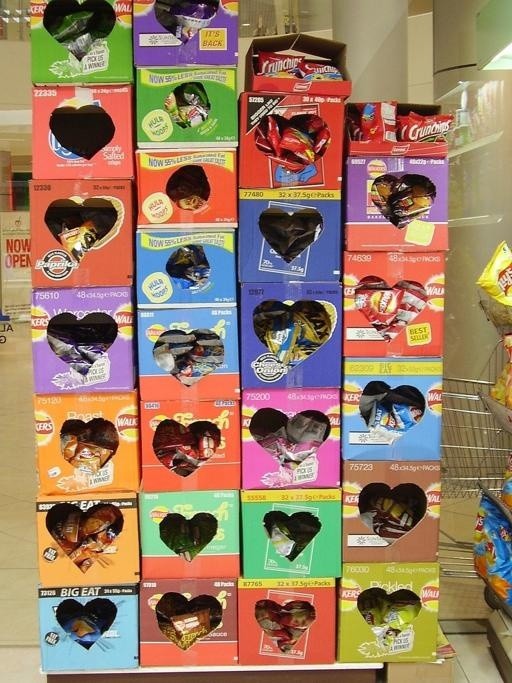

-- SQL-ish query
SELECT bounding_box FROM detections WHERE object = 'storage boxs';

[29,179,135,289]
[344,156,451,252]
[135,306,241,401]
[240,389,342,491]
[134,149,240,228]
[29,0,135,87]
[32,391,141,497]
[341,461,442,563]
[134,228,239,307]
[134,66,240,151]
[342,356,444,461]
[132,0,239,68]
[240,487,343,581]
[239,281,342,390]
[270,122,272,133]
[139,400,240,492]
[237,90,346,192]
[244,32,352,101]
[34,495,141,590]
[341,250,446,358]
[236,191,344,285]
[344,101,449,158]
[138,489,242,579]
[29,287,135,395]
[59,136,63,139]
[137,579,238,667]
[336,562,441,664]
[236,578,337,668]
[31,87,136,179]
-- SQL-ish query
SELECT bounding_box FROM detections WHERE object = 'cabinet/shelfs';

[473,302,512,683]
